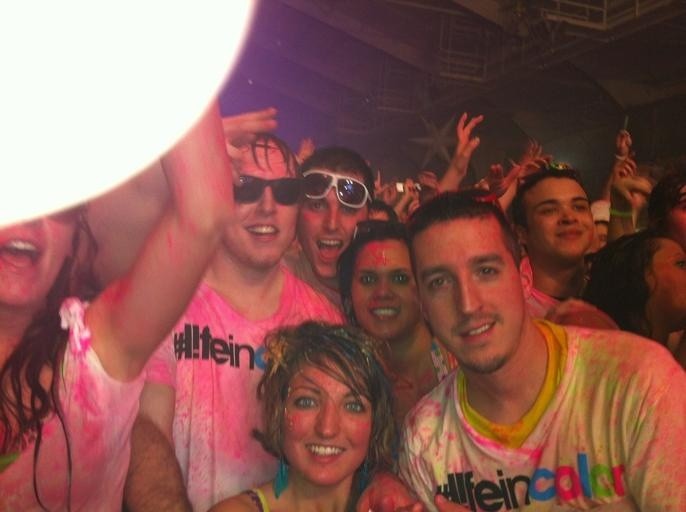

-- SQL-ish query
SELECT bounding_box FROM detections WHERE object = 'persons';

[2,90,239,512]
[71,97,686,512]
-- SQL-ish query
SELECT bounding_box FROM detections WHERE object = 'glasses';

[516,160,578,189]
[230,174,300,205]
[302,172,373,208]
